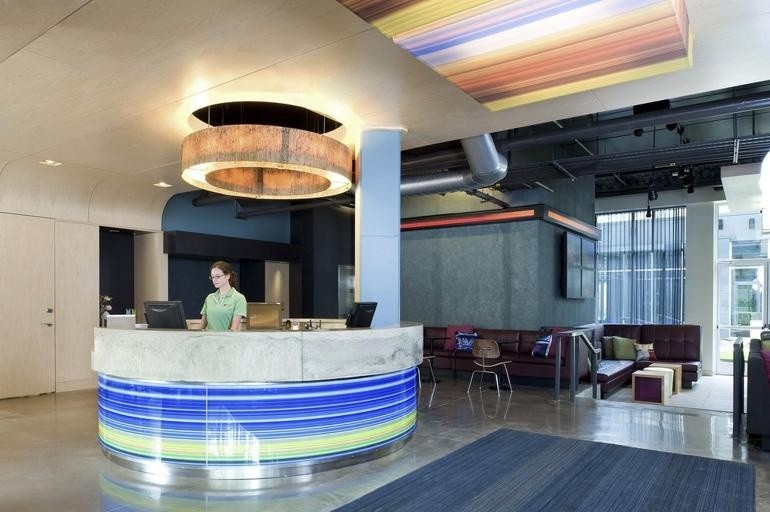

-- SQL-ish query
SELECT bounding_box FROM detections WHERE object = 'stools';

[631,370,670,405]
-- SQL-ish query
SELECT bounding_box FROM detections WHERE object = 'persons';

[196,260,247,331]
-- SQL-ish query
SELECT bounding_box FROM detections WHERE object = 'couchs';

[415,322,703,399]
[747,339,770,453]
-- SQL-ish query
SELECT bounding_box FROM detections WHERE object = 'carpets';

[328,426,756,512]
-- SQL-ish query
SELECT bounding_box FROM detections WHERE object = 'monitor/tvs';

[143,301,189,329]
[345,302,378,328]
[246,302,283,329]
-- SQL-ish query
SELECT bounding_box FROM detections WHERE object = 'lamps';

[648,126,658,201]
[180,104,353,200]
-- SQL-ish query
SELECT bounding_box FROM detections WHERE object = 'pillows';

[760,339,770,383]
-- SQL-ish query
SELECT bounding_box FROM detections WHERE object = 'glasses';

[208,274,224,280]
